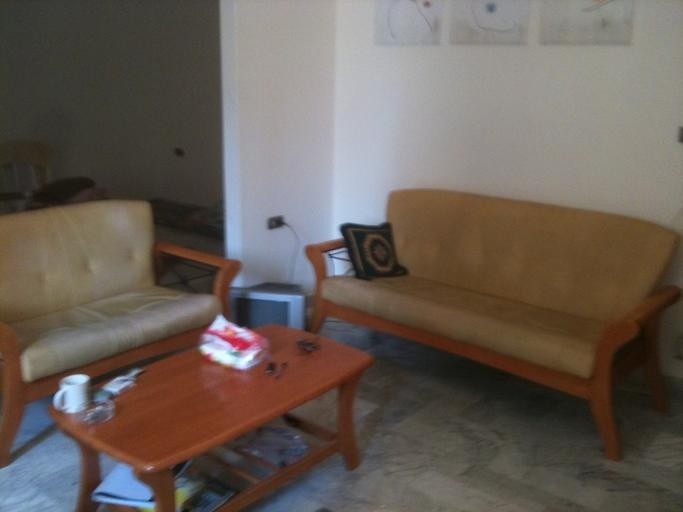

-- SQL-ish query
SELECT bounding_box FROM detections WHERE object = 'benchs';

[2,197,242,468]
[303,187,682,463]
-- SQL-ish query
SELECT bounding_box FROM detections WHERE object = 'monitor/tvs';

[231,282,312,331]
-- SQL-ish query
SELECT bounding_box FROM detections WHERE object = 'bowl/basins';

[77,400,115,425]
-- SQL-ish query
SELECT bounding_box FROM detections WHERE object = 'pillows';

[338,218,411,284]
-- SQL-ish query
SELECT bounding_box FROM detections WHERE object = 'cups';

[52,373,91,414]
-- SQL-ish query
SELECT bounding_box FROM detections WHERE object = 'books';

[143,469,238,511]
[89,458,192,509]
